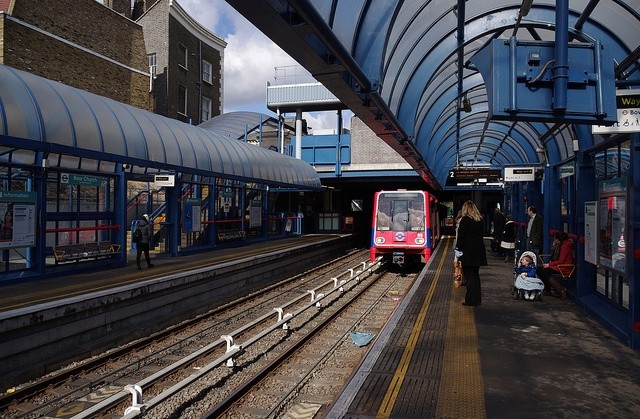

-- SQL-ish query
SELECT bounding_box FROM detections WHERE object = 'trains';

[369,187,451,265]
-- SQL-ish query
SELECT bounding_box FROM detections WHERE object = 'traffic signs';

[504,167,535,182]
[154,174,175,187]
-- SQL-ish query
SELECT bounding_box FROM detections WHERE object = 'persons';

[524,206,543,257]
[493,208,506,258]
[136,214,154,271]
[500,214,515,263]
[514,255,537,301]
[452,210,467,288]
[538,231,578,301]
[454,200,488,306]
[1,211,13,242]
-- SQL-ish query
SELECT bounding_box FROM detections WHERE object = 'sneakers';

[530,293,535,300]
[524,293,529,299]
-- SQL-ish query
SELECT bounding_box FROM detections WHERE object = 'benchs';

[539,254,575,280]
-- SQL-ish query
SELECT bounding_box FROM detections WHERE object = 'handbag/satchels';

[454,256,463,289]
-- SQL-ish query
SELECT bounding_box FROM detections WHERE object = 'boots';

[548,277,568,300]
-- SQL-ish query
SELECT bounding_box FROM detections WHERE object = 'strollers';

[510,239,543,301]
